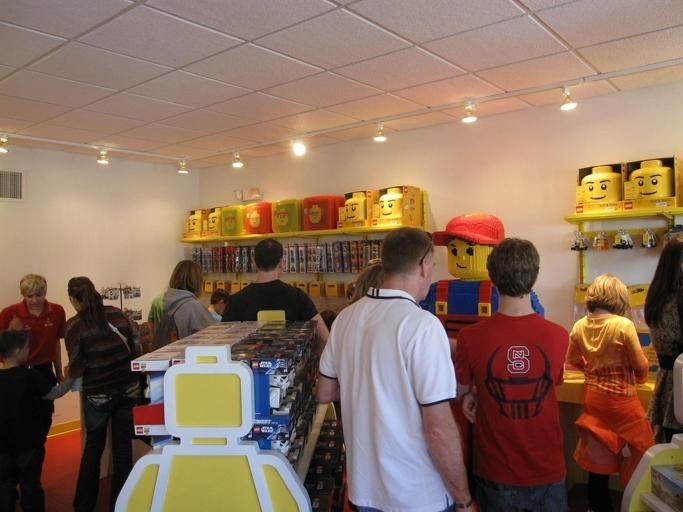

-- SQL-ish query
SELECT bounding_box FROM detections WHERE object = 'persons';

[62,276,142,512]
[222,238,329,347]
[565,273,657,512]
[313,227,472,511]
[644,239,683,443]
[1,331,75,511]
[1,273,67,436]
[156,259,216,351]
[349,259,385,304]
[320,306,336,333]
[147,294,163,352]
[452,237,570,512]
[207,288,230,322]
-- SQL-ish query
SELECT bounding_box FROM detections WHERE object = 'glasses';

[419,230,435,266]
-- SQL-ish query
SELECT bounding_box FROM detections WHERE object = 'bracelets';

[455,498,473,509]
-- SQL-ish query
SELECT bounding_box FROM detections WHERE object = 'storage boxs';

[219,193,345,237]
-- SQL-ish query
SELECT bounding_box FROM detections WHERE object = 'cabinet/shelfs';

[107,317,346,511]
[616,351,683,512]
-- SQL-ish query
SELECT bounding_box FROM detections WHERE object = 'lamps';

[0,82,582,176]
